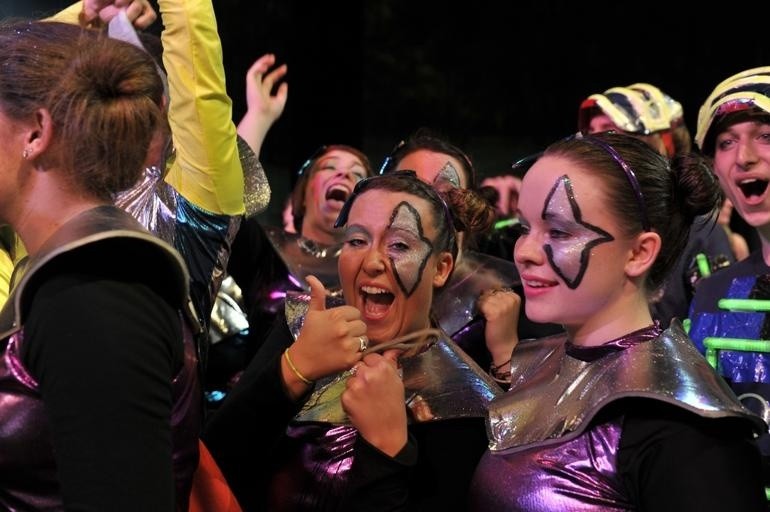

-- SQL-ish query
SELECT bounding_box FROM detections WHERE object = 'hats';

[692,65,770,156]
[574,80,688,137]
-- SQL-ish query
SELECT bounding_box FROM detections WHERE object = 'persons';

[1,1,769,512]
